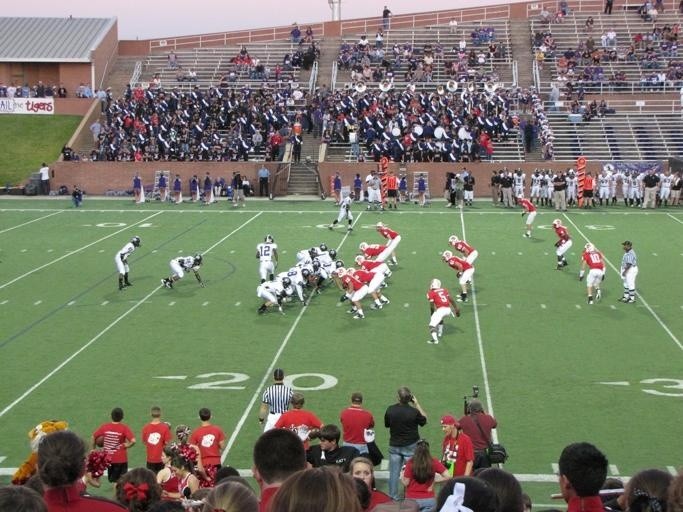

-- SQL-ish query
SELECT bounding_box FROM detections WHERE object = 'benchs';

[98,25,321,164]
[329,15,525,163]
[526,1,683,161]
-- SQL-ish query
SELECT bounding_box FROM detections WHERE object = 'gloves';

[297,424,312,442]
[364,429,375,443]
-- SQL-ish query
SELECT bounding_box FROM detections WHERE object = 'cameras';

[441,460,451,469]
[463,386,479,416]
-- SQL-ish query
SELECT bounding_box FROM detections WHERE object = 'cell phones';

[410,396,414,403]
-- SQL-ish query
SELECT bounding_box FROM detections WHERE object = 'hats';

[621,240,631,246]
[351,392,362,403]
[274,368,284,380]
[441,415,461,428]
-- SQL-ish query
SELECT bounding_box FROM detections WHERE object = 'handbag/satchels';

[486,443,509,464]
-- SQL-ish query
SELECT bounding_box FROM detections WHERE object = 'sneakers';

[523,233,532,239]
[118,285,127,291]
[347,259,399,320]
[165,282,172,289]
[160,279,166,286]
[552,259,636,305]
[426,339,439,345]
[256,310,267,317]
[124,282,133,286]
[455,297,469,302]
[456,293,461,298]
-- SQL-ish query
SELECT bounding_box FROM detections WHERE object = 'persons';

[0,365,683,512]
[515,192,537,238]
[306,0,682,213]
[112,234,142,291]
[1,21,321,209]
[250,217,481,346]
[552,217,574,271]
[158,251,207,290]
[327,190,357,232]
[619,239,641,304]
[576,242,607,305]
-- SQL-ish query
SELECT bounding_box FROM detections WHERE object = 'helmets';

[553,219,562,229]
[491,166,672,179]
[449,236,458,245]
[264,235,273,244]
[130,235,140,248]
[584,242,595,254]
[193,254,201,266]
[283,223,383,289]
[442,251,452,261]
[429,278,441,291]
